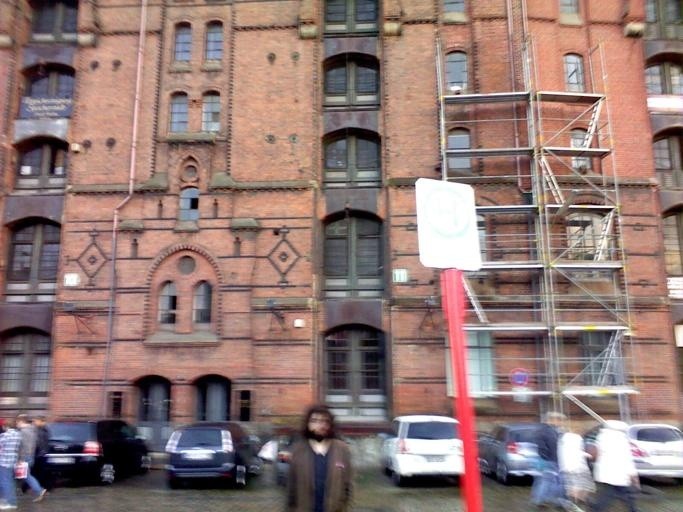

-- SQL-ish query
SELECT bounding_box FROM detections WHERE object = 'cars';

[476,422,564,487]
[28,418,150,487]
[582,419,683,481]
[163,420,264,489]
[376,413,474,486]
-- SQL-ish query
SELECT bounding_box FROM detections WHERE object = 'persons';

[0,418,23,511]
[549,427,596,506]
[31,414,55,492]
[592,417,643,512]
[529,410,575,511]
[13,413,48,504]
[287,404,354,511]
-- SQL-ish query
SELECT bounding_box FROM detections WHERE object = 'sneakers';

[1,488,49,509]
